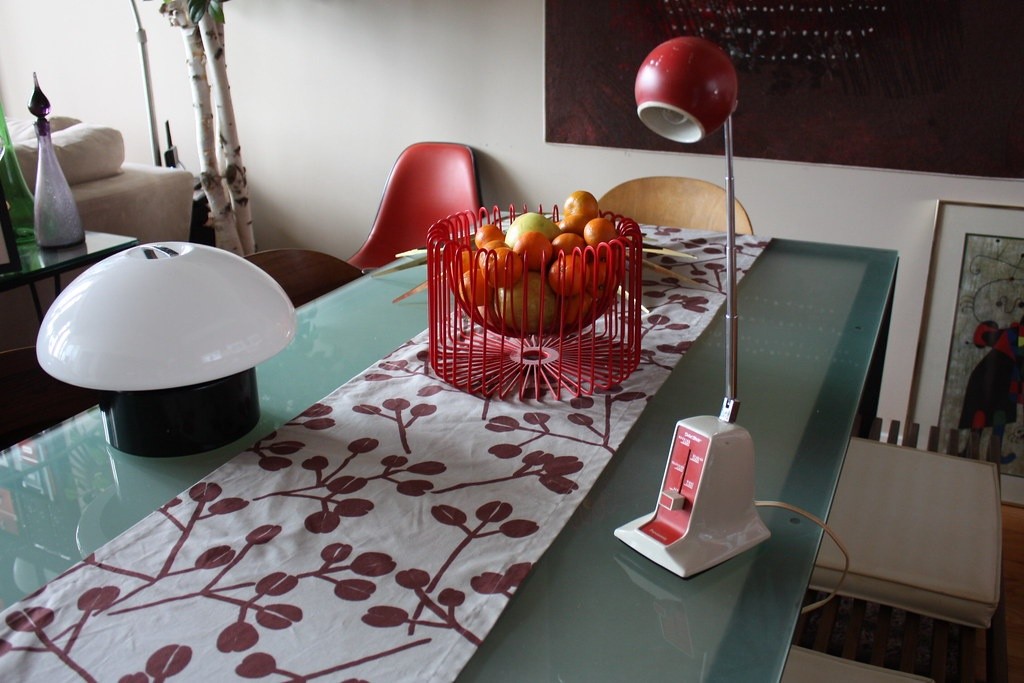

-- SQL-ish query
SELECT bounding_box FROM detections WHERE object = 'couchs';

[0,116,193,354]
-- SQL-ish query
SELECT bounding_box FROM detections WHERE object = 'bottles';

[0,104,37,245]
[27,71,86,250]
[0,177,23,278]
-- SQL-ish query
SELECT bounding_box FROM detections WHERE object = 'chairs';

[0,345,100,452]
[594,176,753,243]
[243,140,486,312]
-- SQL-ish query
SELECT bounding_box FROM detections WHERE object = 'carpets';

[791,544,1008,683]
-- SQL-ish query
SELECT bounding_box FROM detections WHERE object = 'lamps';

[613,35,776,577]
[36,241,298,459]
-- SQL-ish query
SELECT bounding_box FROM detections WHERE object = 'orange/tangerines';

[457,191,619,330]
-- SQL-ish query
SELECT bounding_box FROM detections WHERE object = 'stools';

[780,432,1001,683]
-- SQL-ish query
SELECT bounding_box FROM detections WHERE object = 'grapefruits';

[497,275,558,327]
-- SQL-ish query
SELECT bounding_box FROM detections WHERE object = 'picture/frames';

[904,198,1024,510]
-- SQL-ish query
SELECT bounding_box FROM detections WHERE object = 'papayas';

[506,212,561,248]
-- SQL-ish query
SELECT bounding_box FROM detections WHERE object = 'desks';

[0,210,900,683]
[0,227,140,329]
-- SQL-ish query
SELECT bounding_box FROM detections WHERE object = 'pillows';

[5,116,124,197]
